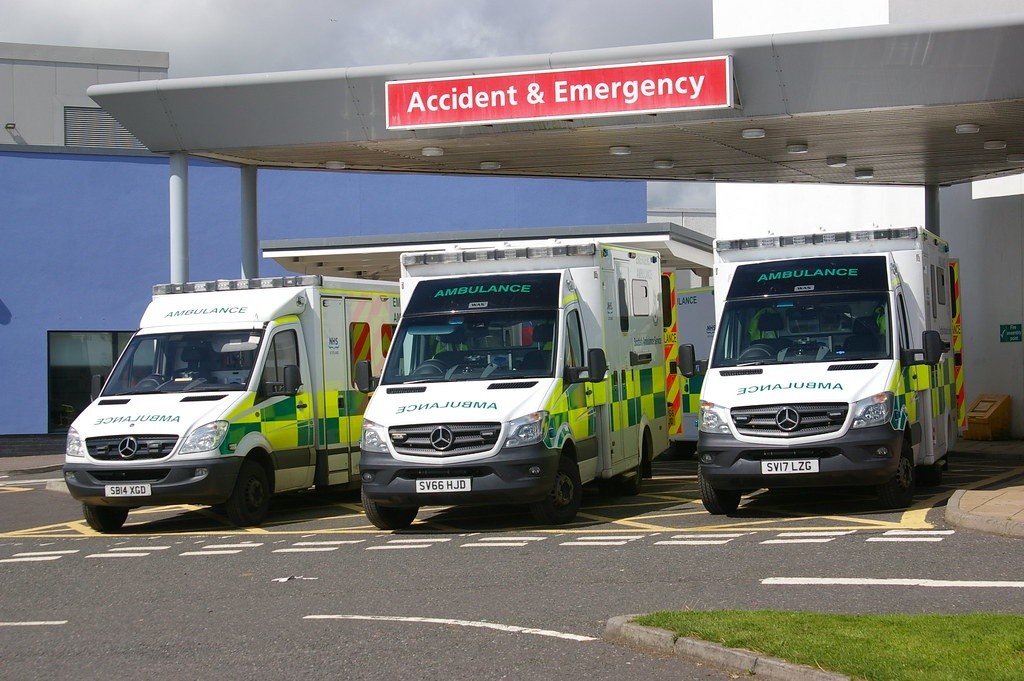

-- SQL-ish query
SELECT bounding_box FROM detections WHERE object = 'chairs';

[751,314,791,353]
[523,324,553,369]
[843,316,885,352]
[175,348,210,381]
[432,327,467,368]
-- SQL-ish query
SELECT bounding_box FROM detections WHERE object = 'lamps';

[653,161,674,169]
[325,161,344,169]
[610,147,630,156]
[828,158,848,168]
[1006,154,1024,162]
[742,129,766,138]
[787,145,807,155]
[985,141,1005,150]
[421,147,444,155]
[696,174,714,180]
[955,123,980,133]
[855,171,874,178]
[480,162,500,169]
[6,123,16,129]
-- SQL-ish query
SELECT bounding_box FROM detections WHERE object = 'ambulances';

[62,274,404,531]
[695,224,969,516]
[359,237,692,538]
